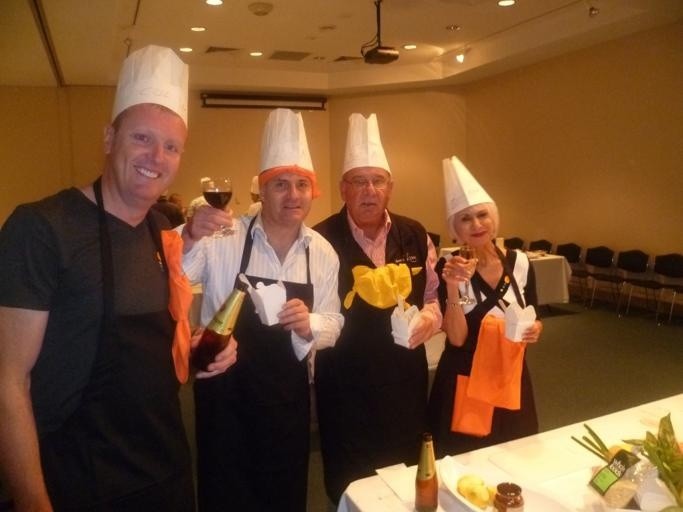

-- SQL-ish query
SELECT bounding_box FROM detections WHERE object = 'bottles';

[414,432,439,511]
[489,479,525,511]
[187,280,253,386]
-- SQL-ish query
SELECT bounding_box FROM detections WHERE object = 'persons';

[167,194,188,219]
[242,193,263,217]
[0,104,238,511]
[306,167,443,507]
[151,191,183,230]
[425,203,542,461]
[177,170,346,511]
[185,176,213,216]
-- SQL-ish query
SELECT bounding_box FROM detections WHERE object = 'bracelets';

[446,299,460,307]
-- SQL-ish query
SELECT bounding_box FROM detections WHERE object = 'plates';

[442,466,510,512]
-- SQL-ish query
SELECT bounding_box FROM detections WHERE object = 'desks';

[338,387,683,512]
[441,246,572,308]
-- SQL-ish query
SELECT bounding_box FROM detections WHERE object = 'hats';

[109,44,189,128]
[342,112,391,178]
[442,155,493,220]
[257,107,314,171]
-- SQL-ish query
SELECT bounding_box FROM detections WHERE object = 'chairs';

[502,239,683,324]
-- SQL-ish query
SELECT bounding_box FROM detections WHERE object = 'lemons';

[465,482,489,509]
[456,473,484,498]
[487,484,497,505]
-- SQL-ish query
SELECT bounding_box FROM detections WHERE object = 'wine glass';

[454,242,480,306]
[200,175,235,239]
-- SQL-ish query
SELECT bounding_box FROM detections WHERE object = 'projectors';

[365,46,399,64]
